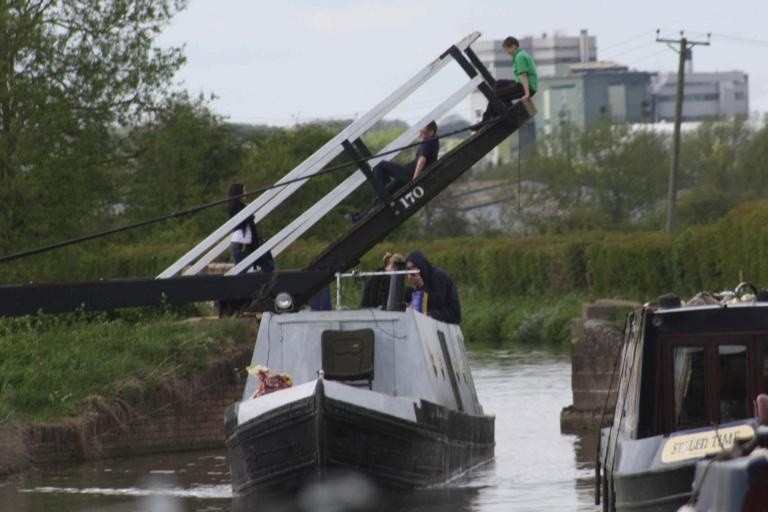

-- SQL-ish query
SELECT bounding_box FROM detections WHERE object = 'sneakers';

[344,212,361,223]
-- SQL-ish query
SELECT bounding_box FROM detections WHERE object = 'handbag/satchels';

[231,225,251,244]
[411,290,428,315]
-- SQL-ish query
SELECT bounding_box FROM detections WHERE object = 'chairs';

[318,329,375,391]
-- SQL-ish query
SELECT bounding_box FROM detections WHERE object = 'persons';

[375,121,439,196]
[470,38,538,131]
[360,252,407,307]
[404,251,462,324]
[229,184,254,274]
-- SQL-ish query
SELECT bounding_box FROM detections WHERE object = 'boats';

[225,268,496,503]
[597,281,768,512]
